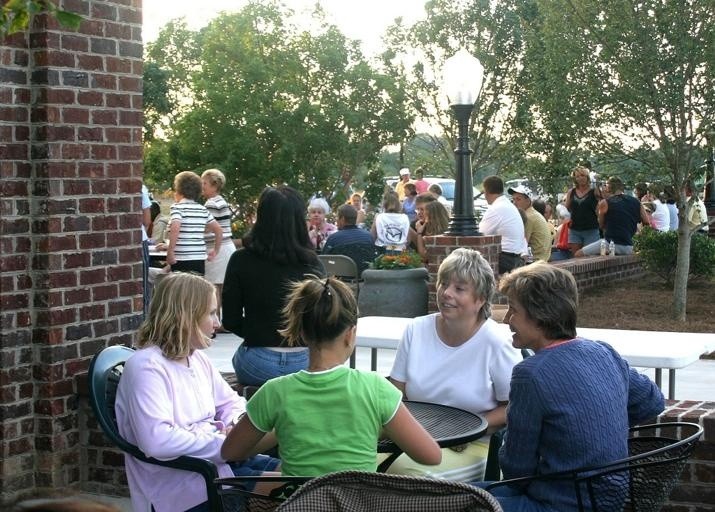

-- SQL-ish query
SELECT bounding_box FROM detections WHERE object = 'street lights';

[439,45,488,236]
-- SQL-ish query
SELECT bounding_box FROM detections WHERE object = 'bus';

[504,173,606,206]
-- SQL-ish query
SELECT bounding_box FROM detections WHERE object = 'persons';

[221,187,327,385]
[141,183,153,322]
[148,199,171,246]
[167,171,223,279]
[459,259,665,511]
[219,274,441,502]
[112,270,287,511]
[373,246,523,484]
[153,241,170,252]
[200,169,237,334]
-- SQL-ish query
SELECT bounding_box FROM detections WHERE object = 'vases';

[358,269,428,320]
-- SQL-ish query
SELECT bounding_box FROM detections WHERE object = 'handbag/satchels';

[558,220,571,249]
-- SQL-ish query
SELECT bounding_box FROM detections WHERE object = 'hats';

[399,167,410,176]
[506,185,533,200]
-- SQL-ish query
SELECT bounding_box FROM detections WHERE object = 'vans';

[387,176,488,224]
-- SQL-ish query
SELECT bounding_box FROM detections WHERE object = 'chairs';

[88,344,221,511]
[312,253,365,312]
[484,423,704,510]
[213,475,319,511]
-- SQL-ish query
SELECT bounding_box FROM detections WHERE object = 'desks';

[348,309,715,406]
[377,401,490,474]
[143,235,169,262]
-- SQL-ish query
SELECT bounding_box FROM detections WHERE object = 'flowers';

[362,243,427,272]
